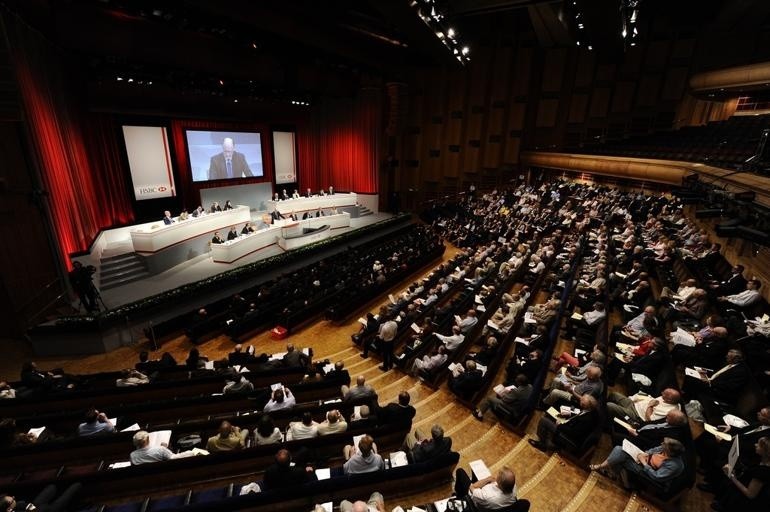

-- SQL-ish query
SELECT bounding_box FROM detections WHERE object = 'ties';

[227,159,231,178]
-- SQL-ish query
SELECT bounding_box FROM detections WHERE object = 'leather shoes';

[379,366,388,371]
[472,407,483,420]
[528,439,546,452]
[360,354,368,358]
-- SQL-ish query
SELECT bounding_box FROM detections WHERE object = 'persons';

[446,168,769,509]
[0,342,532,512]
[209,137,254,180]
[123,185,446,341]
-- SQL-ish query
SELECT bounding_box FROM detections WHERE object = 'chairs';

[429,175,670,247]
[151,192,347,262]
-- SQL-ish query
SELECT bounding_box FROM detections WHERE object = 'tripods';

[78,279,109,316]
